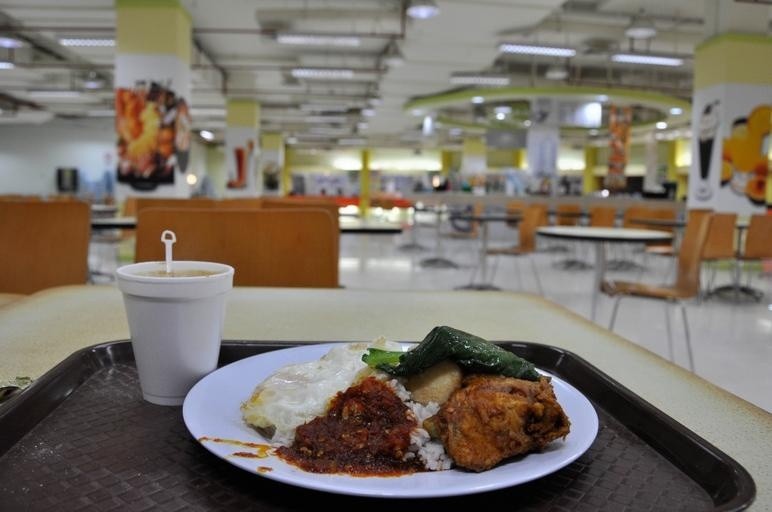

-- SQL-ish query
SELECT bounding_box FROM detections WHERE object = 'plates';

[182,341,602,499]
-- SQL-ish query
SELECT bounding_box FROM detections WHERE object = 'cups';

[117,260,234,406]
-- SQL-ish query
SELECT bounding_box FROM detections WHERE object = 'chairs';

[587,205,617,272]
[736,213,770,303]
[699,212,742,305]
[600,211,714,376]
[431,198,550,300]
[623,206,652,269]
[646,207,679,275]
[0,191,344,295]
[555,205,578,268]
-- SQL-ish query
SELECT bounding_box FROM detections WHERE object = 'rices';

[372,372,453,470]
